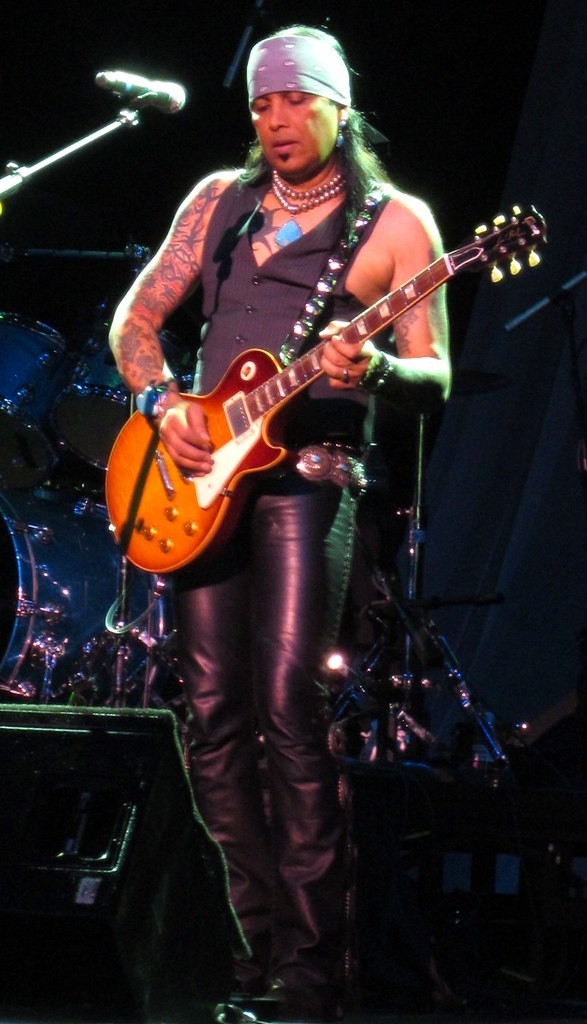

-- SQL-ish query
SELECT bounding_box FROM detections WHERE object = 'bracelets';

[369,351,395,392]
[135,380,178,418]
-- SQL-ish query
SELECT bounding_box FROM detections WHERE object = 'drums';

[48,319,196,471]
[0,310,92,492]
[0,487,165,708]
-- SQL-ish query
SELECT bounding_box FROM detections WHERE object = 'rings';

[342,369,348,380]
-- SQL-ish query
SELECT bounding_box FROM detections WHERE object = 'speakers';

[0,700,253,1024]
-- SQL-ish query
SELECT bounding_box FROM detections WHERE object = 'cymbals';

[451,367,512,397]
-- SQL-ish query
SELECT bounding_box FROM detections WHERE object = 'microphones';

[96,71,187,113]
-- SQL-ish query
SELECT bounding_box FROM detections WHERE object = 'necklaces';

[272,169,348,214]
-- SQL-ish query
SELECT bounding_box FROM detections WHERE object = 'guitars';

[102,201,553,581]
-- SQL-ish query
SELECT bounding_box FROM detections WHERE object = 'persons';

[104,29,454,1024]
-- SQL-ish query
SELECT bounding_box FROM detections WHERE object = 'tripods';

[331,410,515,790]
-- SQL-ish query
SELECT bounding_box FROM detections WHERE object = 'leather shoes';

[213,978,327,1024]
